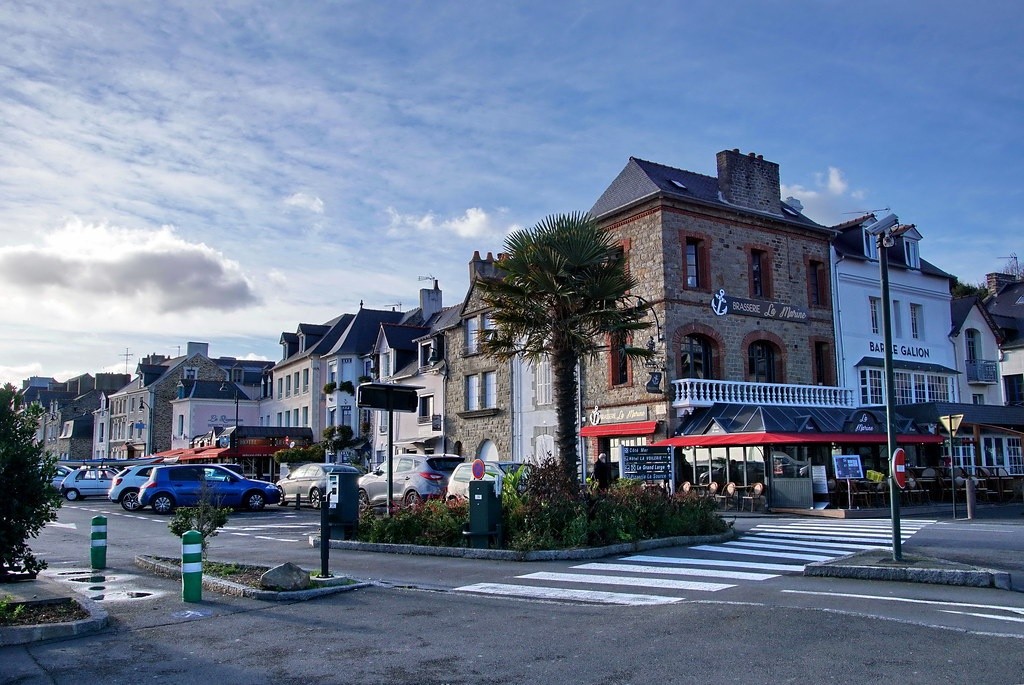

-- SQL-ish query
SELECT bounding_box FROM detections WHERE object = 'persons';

[595,453,609,489]
[724,460,743,486]
[681,454,693,485]
[921,463,935,478]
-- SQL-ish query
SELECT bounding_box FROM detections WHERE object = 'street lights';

[867,212,912,561]
[135,398,152,456]
[221,381,238,464]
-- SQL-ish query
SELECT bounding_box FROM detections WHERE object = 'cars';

[36,463,94,495]
[277,463,365,512]
[59,467,121,501]
[202,463,245,483]
[137,464,281,516]
[446,461,539,510]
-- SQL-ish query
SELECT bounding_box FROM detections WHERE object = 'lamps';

[427,347,441,362]
[171,434,175,441]
[623,293,662,342]
[182,434,186,441]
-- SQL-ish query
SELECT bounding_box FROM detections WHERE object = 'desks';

[732,486,751,512]
[691,485,706,494]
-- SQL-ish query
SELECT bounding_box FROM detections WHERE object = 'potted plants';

[273,445,324,481]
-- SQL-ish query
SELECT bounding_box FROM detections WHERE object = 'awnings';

[581,421,660,438]
[392,436,439,444]
[649,434,944,446]
[156,445,307,460]
[353,438,373,450]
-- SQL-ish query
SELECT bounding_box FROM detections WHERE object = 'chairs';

[832,467,1014,507]
[100,473,107,479]
[701,482,718,512]
[715,482,735,512]
[678,481,690,492]
[741,483,763,513]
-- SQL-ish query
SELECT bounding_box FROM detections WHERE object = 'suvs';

[106,464,164,512]
[358,452,465,511]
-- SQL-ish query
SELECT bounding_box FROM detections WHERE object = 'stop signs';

[891,447,910,490]
